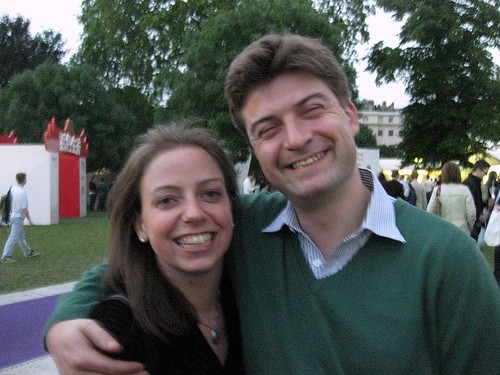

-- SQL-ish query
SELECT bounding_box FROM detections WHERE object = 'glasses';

[477,166,487,175]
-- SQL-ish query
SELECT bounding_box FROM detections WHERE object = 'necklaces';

[193,304,223,344]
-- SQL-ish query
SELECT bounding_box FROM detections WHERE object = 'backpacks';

[0,186,12,224]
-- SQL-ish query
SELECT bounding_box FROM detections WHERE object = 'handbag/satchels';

[434,185,442,216]
[406,183,416,206]
[484,205,500,246]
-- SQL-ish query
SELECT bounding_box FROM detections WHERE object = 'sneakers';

[3,257,16,263]
[26,250,39,257]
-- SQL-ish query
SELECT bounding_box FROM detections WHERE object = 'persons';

[243,159,499,283]
[44,32,500,374]
[89,176,98,211]
[87,119,242,374]
[97,176,107,210]
[0,173,41,263]
[109,176,116,189]
